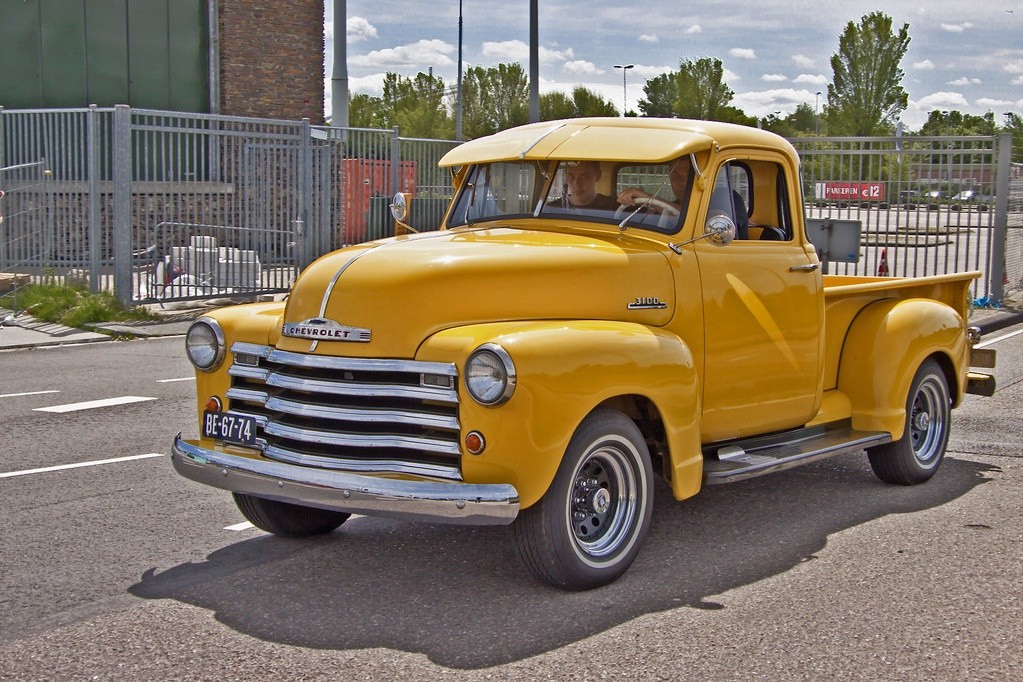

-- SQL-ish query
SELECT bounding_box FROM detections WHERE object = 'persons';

[615,154,749,240]
[544,159,621,216]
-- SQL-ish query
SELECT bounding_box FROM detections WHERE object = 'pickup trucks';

[168,118,995,590]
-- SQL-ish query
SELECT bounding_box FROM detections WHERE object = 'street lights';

[613,64,634,116]
[816,91,821,137]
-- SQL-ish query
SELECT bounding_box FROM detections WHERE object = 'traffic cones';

[878,249,889,276]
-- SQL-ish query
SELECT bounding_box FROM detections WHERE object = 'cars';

[923,191,945,201]
[898,190,916,203]
[952,191,980,204]
